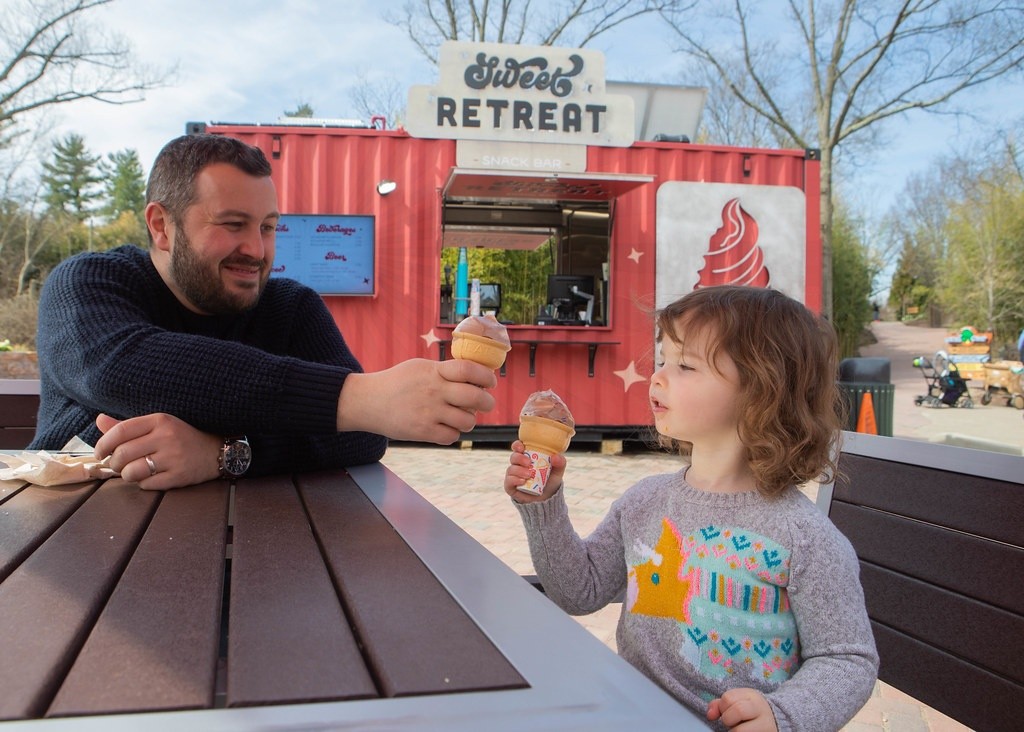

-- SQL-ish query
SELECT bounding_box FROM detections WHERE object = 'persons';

[23,134,499,494]
[505,284,883,732]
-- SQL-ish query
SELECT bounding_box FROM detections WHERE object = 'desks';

[1,447,713,730]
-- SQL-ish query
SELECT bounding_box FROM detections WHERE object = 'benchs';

[813,428,1023,732]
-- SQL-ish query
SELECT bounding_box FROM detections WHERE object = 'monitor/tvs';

[547,274,594,306]
[268,213,376,298]
[467,283,501,309]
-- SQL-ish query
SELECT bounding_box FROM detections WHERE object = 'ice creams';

[450,310,511,369]
[516,389,578,497]
[694,198,771,290]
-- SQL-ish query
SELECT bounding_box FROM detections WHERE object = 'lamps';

[376,180,397,196]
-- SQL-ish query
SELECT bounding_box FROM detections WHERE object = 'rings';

[145,455,157,477]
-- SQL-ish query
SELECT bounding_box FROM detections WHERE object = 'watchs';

[217,431,254,483]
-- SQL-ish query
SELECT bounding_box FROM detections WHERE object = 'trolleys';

[981,358,1024,410]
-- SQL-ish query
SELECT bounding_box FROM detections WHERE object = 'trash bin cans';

[835,356,895,438]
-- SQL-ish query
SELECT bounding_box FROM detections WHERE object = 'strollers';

[912,350,974,409]
